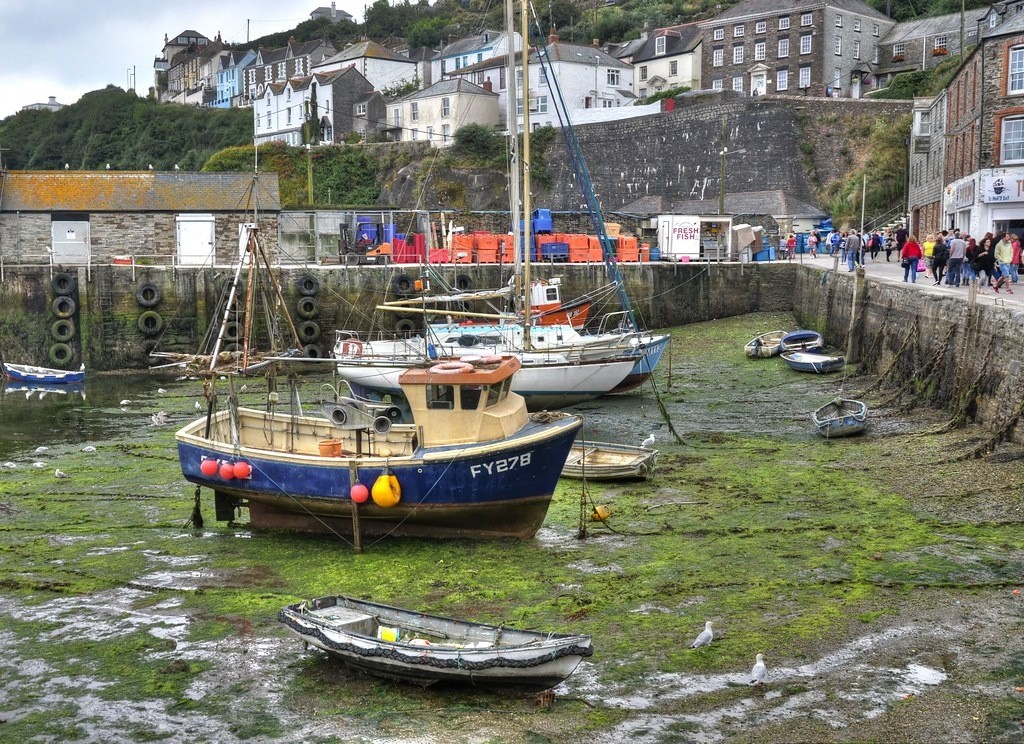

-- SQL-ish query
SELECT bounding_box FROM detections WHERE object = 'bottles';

[428,343,436,358]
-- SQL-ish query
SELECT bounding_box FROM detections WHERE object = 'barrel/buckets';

[318,440,342,456]
[650,248,659,261]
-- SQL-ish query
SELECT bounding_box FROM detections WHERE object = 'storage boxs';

[795,221,835,253]
[354,209,638,264]
[732,223,775,261]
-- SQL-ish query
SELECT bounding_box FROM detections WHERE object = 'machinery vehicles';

[338,221,392,266]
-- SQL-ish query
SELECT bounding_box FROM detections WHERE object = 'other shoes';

[992,279,998,282]
[976,285,980,293]
[991,286,999,293]
[1007,290,1013,294]
[984,290,988,294]
[932,281,975,288]
[987,283,993,286]
[1009,281,1017,285]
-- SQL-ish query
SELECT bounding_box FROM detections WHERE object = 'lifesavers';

[135,282,165,367]
[295,273,323,365]
[393,272,417,339]
[452,274,474,315]
[219,275,244,352]
[47,272,76,366]
[459,354,503,365]
[342,337,364,360]
[520,307,542,316]
[428,362,475,374]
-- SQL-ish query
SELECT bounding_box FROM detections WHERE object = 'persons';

[779,223,1024,296]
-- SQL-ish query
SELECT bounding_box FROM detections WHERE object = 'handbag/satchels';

[868,239,873,246]
[969,258,981,268]
[902,259,909,268]
[916,259,926,271]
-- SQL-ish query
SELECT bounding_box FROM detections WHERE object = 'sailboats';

[339,1,672,395]
[331,1,645,412]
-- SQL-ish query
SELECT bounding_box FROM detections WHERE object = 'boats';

[459,277,592,331]
[744,330,788,359]
[149,92,585,541]
[277,594,593,694]
[780,350,846,374]
[812,397,868,438]
[4,377,86,401]
[559,440,659,482]
[4,362,86,384]
[781,329,824,353]
